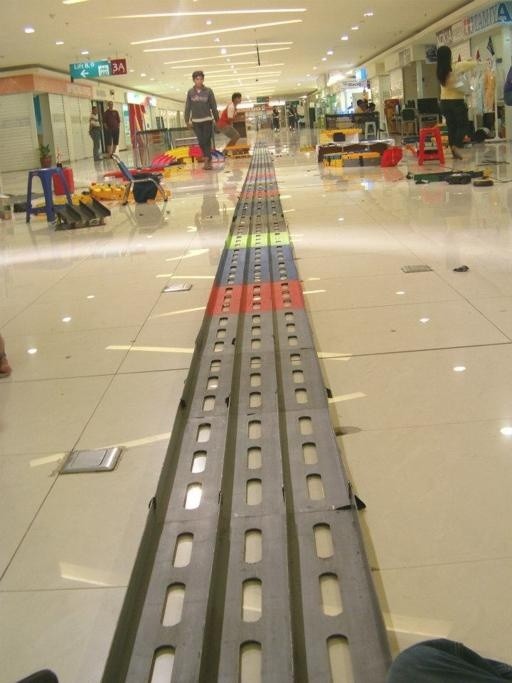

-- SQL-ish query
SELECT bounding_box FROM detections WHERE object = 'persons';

[435,44,480,159]
[503,65,512,105]
[89,106,105,161]
[185,70,221,169]
[271,108,280,131]
[103,100,120,158]
[217,92,242,154]
[0,335,14,381]
[287,104,297,130]
[386,637,511,682]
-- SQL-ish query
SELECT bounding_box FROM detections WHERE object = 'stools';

[416,128,446,166]
[364,121,379,140]
[25,167,74,223]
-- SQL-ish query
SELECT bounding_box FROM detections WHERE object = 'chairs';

[110,153,169,207]
[401,109,420,139]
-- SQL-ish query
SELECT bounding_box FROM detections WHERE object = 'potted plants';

[38,145,52,167]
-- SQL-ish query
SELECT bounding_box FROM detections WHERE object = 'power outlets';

[399,264,434,275]
[56,445,123,475]
[160,280,194,294]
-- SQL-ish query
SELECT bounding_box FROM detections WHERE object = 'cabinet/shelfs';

[465,61,504,143]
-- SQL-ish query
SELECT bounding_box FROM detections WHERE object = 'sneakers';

[450,145,465,160]
[203,160,215,171]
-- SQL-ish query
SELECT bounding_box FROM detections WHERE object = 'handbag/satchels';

[453,72,475,97]
